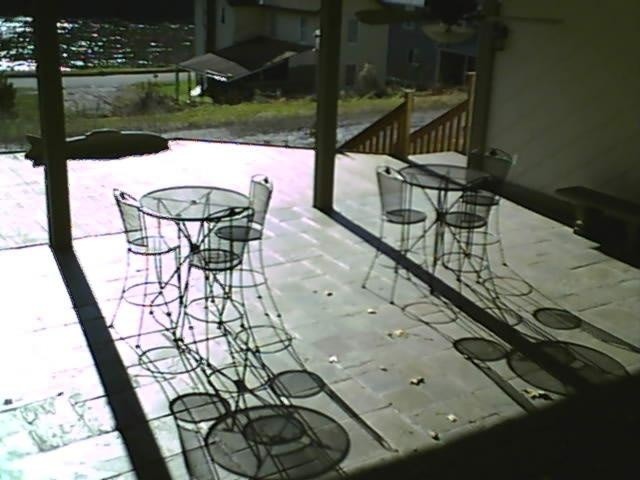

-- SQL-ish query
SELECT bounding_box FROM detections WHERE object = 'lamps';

[421,17,476,44]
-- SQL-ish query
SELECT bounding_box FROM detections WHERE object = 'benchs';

[554,185,640,253]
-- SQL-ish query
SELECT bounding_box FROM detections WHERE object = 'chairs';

[108,174,273,348]
[361,146,513,305]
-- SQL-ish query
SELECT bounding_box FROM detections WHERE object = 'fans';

[354,0,566,27]
